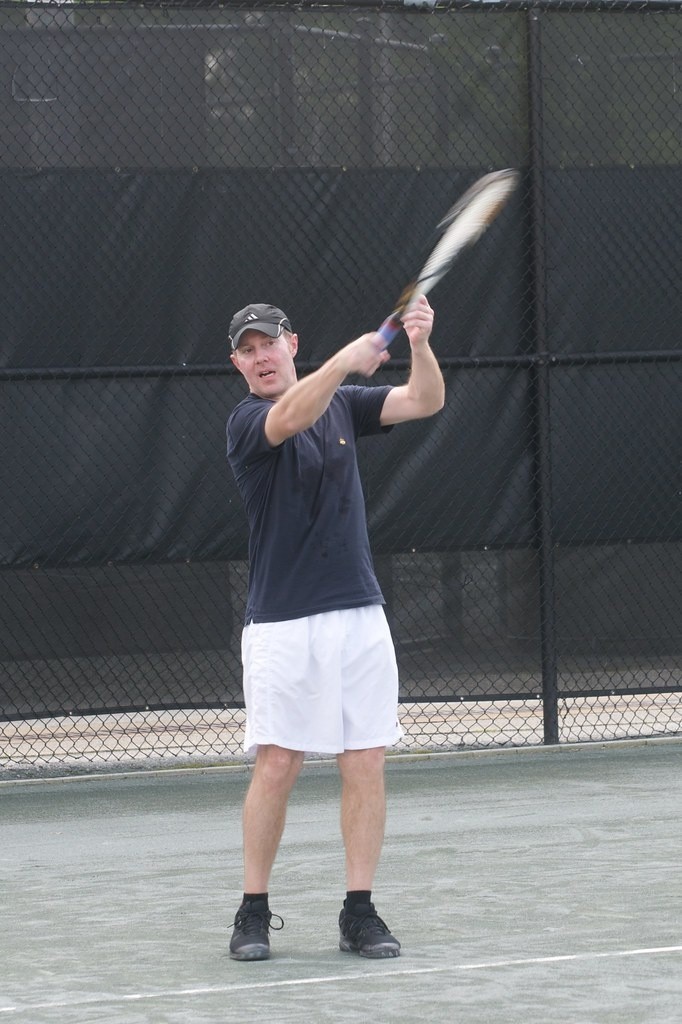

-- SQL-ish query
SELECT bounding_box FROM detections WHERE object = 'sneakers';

[228,901,271,960]
[338,902,401,959]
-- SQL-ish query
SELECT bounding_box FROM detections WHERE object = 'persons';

[220,290,446,963]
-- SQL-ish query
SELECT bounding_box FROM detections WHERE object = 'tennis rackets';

[377,165,522,345]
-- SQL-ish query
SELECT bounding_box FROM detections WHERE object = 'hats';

[226,303,292,351]
